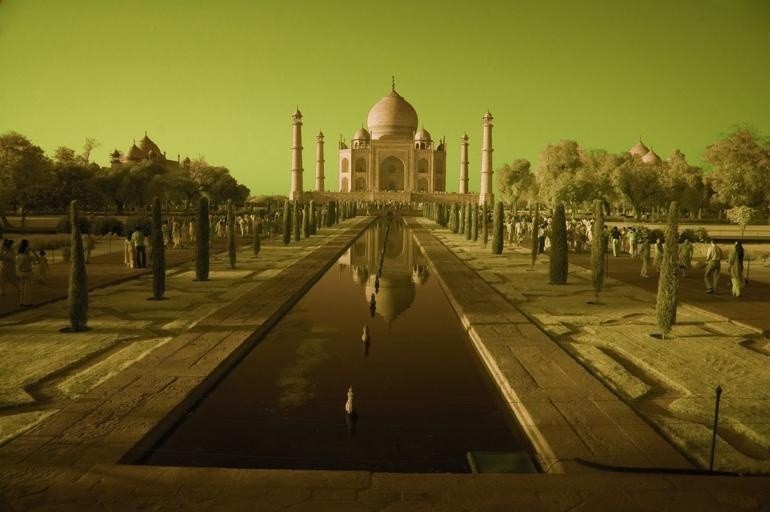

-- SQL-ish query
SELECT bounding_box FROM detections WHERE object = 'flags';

[0,238,49,307]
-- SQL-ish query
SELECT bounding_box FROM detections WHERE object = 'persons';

[124,225,152,269]
[605,223,662,279]
[161,217,197,251]
[357,198,423,211]
[727,240,745,298]
[506,210,594,253]
[209,212,273,240]
[678,238,694,279]
[81,231,99,264]
[702,238,726,295]
